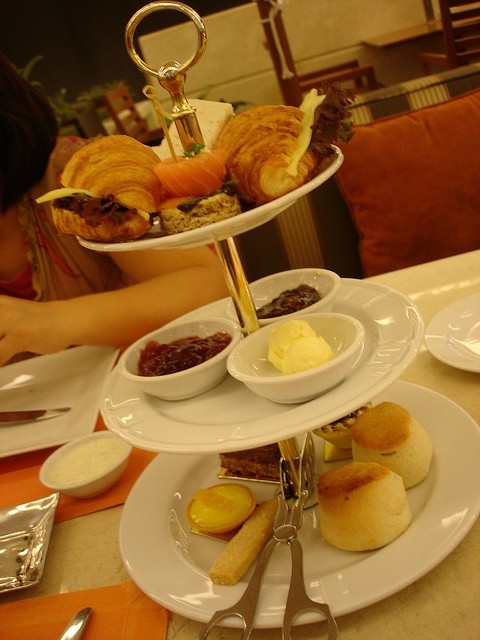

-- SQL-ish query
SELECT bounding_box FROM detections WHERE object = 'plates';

[0,343,123,459]
[0,490,61,594]
[73,142,345,255]
[97,271,424,458]
[117,376,480,631]
[423,290,480,374]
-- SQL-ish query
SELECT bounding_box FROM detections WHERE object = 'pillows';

[332,90,480,276]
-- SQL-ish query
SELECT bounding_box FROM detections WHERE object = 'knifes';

[0,406,72,427]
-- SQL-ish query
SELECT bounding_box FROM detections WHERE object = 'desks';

[362,20,452,77]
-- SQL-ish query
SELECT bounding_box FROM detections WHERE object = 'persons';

[0,56,232,371]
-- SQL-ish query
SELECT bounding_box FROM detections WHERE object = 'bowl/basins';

[225,311,367,407]
[116,316,242,404]
[38,429,133,501]
[247,265,343,328]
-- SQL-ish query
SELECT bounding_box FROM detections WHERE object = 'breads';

[49,136,160,243]
[218,444,281,480]
[316,462,411,553]
[350,400,434,489]
[214,105,316,205]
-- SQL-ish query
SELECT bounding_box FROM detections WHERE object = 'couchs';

[232,62,480,281]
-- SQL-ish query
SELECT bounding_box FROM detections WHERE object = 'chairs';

[420,0,480,73]
[102,82,168,143]
[254,0,384,106]
[75,100,110,140]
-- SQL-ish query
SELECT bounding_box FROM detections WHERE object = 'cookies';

[210,497,278,585]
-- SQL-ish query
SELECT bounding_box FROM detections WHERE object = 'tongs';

[198,431,340,640]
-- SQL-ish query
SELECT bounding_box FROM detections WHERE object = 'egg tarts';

[185,482,255,533]
